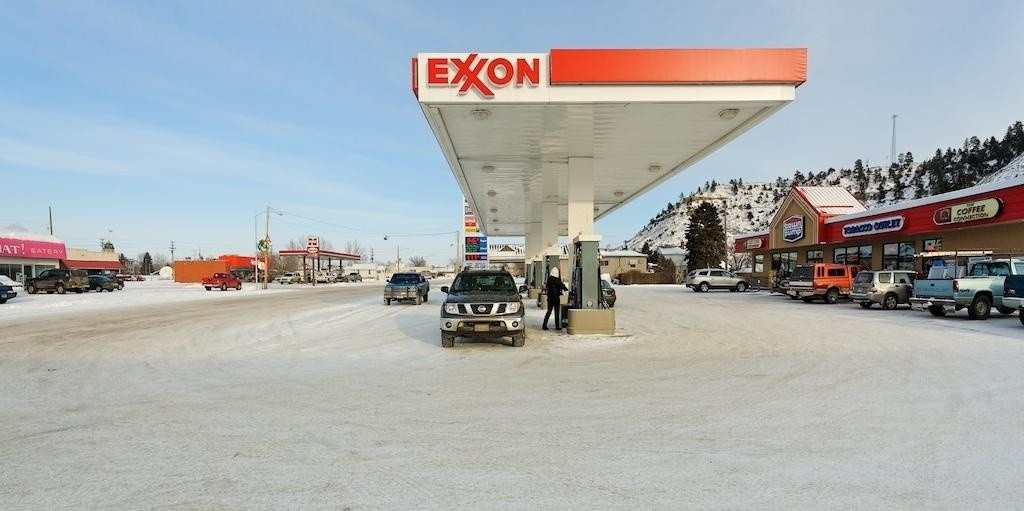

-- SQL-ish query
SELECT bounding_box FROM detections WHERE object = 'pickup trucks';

[201,272,242,291]
[788,262,866,305]
[907,256,1023,320]
[383,272,430,306]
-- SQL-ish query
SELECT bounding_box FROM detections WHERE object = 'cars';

[0,268,146,304]
[600,280,616,308]
[686,268,749,293]
[279,269,363,285]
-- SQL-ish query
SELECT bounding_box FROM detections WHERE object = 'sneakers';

[543,326,562,331]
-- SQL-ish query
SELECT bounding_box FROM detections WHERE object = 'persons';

[541,267,568,330]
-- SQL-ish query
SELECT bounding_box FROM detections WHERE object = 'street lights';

[254,210,284,291]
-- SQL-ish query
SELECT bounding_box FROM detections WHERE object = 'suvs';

[439,266,527,348]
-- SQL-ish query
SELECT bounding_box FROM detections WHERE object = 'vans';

[848,269,921,310]
